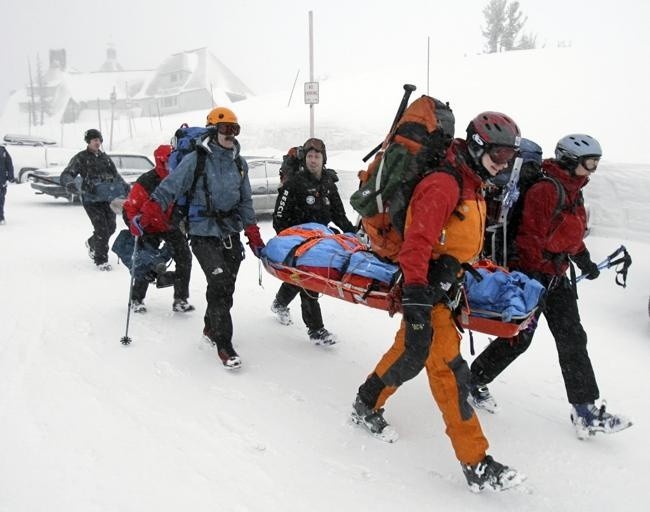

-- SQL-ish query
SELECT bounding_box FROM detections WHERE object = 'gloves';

[66,183,78,194]
[128,214,144,237]
[402,284,436,324]
[244,224,265,258]
[569,250,600,281]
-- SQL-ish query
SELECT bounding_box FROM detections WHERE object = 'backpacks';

[350,97,464,265]
[278,144,308,185]
[486,139,564,267]
[169,124,240,225]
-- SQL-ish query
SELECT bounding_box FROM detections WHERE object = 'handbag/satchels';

[112,230,167,285]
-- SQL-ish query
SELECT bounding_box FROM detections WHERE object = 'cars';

[0,152,299,223]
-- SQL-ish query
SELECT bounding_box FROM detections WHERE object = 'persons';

[0,146,14,224]
[350,111,529,496]
[466,135,632,440]
[60,129,130,271]
[129,108,266,370]
[269,139,357,348]
[122,146,195,314]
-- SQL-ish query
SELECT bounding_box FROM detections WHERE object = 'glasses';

[218,123,240,136]
[579,157,599,171]
[487,145,516,164]
[303,139,325,152]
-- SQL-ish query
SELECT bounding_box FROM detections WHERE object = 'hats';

[303,138,327,166]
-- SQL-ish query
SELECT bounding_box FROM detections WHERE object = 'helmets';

[84,129,103,143]
[206,108,239,134]
[467,111,520,167]
[555,134,602,172]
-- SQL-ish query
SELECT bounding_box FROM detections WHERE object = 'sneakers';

[352,394,399,443]
[462,456,526,493]
[572,404,633,440]
[84,239,95,260]
[129,299,339,368]
[98,261,111,272]
[469,370,501,415]
